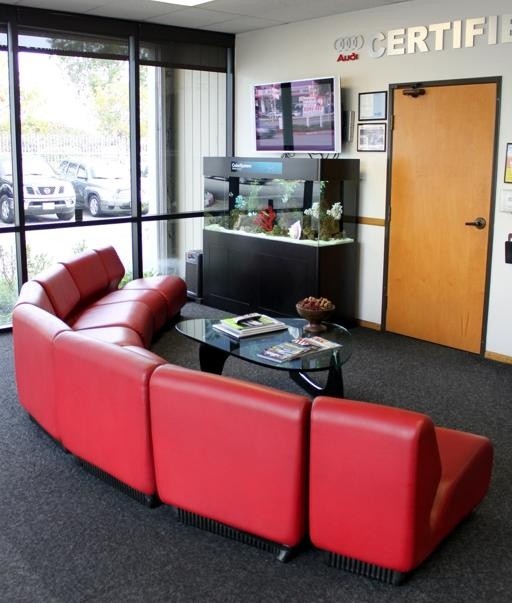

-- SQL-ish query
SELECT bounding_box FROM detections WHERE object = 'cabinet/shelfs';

[201,155,361,332]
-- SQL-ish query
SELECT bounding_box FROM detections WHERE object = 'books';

[257,336,344,364]
[211,312,288,337]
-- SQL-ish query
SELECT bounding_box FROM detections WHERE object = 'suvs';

[0,154,76,223]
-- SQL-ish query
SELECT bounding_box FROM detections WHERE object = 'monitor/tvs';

[252,74,343,153]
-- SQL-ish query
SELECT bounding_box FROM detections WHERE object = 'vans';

[59,159,149,218]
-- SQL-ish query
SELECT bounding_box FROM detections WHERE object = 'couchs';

[54,330,167,508]
[308,396,493,586]
[62,248,166,340]
[13,281,145,347]
[13,305,147,468]
[36,263,153,348]
[149,362,311,561]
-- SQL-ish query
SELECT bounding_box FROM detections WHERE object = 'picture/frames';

[95,246,186,321]
[175,317,354,399]
[358,91,388,120]
[504,143,512,184]
[357,123,386,152]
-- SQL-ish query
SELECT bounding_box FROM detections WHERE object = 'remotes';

[233,312,261,323]
